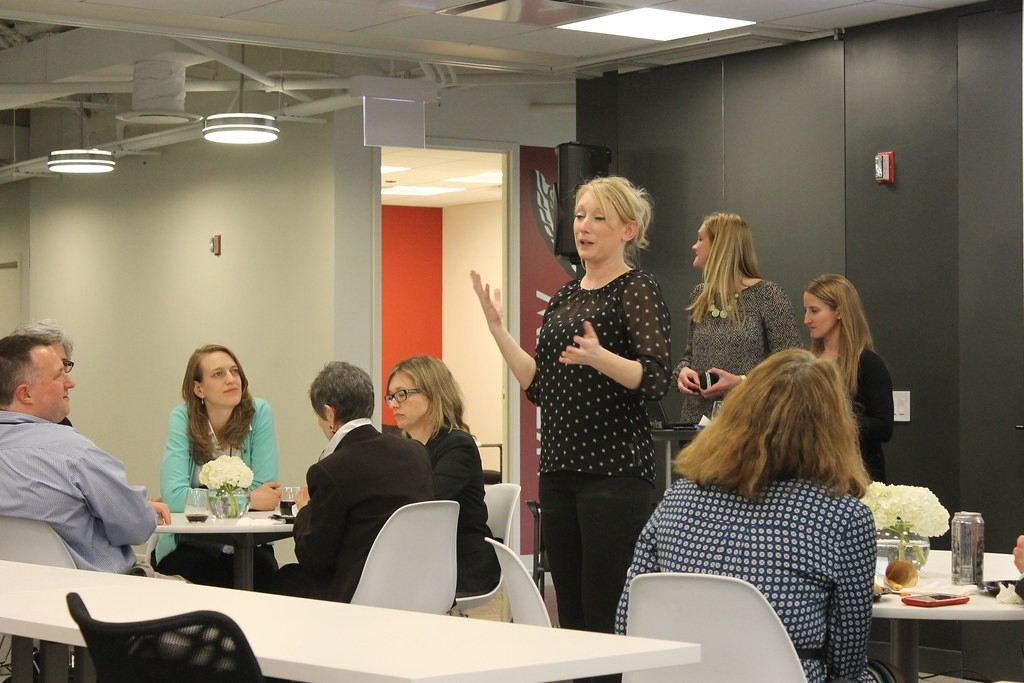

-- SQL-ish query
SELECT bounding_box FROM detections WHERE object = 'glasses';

[384,389,420,406]
[62,360,74,373]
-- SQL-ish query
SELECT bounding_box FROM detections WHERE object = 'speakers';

[553,141,612,254]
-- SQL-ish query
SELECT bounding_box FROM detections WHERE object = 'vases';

[208,485,251,521]
[871,519,930,590]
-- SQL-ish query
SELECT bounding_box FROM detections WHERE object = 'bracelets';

[739,374,747,384]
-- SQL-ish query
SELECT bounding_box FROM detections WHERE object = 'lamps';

[203,45,281,145]
[47,93,115,174]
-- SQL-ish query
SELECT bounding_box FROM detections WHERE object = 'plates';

[269,515,295,524]
[979,579,1024,598]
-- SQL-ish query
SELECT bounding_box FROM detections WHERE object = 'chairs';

[66,591,264,683]
[620,573,808,683]
[483,535,553,628]
[454,483,523,617]
[348,500,461,614]
[0,515,78,651]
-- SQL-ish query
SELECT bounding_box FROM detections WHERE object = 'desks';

[0,560,701,682]
[871,552,1024,683]
[141,513,295,593]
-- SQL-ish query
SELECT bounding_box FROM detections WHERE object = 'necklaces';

[705,272,744,319]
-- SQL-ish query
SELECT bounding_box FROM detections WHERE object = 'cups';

[184,487,210,522]
[279,487,301,518]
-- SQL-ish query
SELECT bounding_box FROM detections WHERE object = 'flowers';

[857,482,952,540]
[200,454,255,493]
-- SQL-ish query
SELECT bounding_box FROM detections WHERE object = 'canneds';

[950,511,984,586]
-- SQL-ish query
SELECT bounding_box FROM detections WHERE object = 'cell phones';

[901,593,970,608]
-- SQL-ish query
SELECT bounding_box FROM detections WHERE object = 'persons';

[0,319,196,588]
[274,362,437,604]
[615,349,904,683]
[469,174,673,683]
[149,344,283,596]
[384,354,504,607]
[1013,534,1024,602]
[668,212,806,434]
[802,274,894,487]
